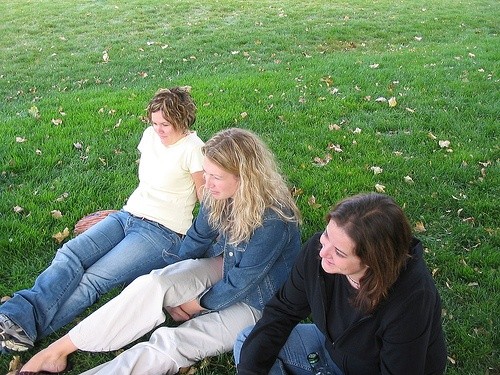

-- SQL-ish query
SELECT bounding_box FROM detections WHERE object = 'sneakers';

[0,314,34,352]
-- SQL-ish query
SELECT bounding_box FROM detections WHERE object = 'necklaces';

[345,275,360,288]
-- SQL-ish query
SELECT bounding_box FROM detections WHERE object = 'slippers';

[15,361,70,375]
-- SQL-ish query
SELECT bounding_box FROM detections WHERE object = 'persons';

[7,128,302,375]
[0,84,206,352]
[234,193,448,375]
